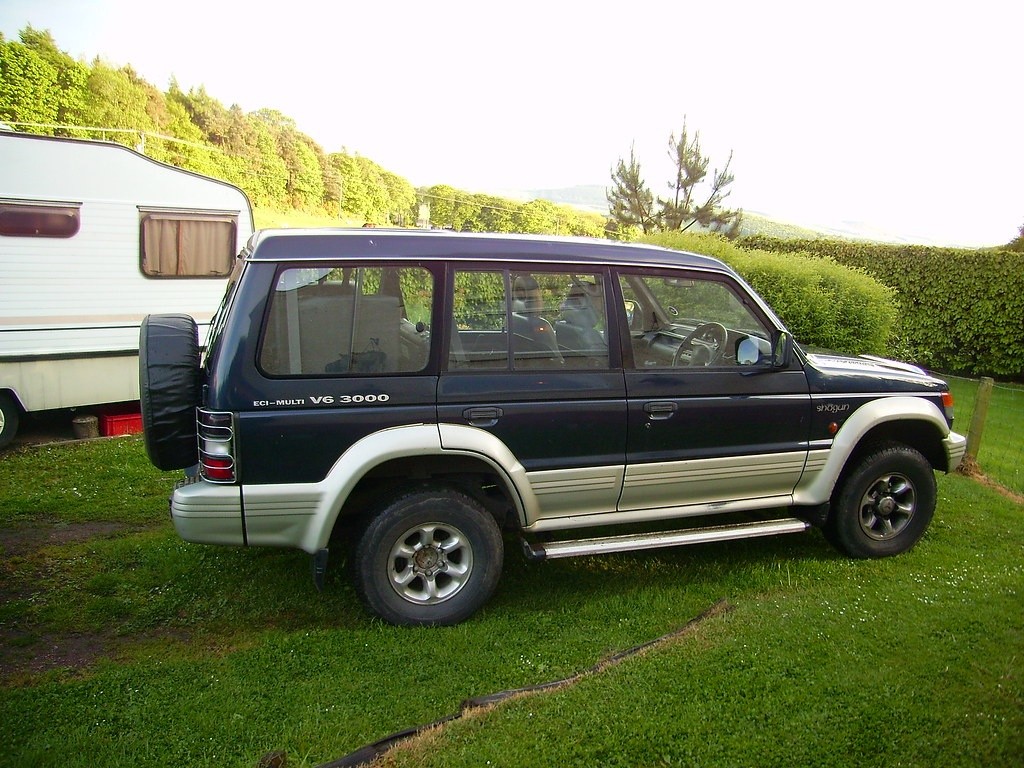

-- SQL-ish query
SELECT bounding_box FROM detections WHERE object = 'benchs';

[399,317,430,372]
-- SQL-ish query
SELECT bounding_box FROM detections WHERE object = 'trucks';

[0,133,256,449]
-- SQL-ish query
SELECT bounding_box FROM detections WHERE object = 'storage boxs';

[99,414,143,437]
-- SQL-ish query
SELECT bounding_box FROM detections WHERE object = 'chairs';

[512,275,565,369]
[556,280,609,369]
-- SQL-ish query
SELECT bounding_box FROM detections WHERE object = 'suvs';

[138,229,967,627]
